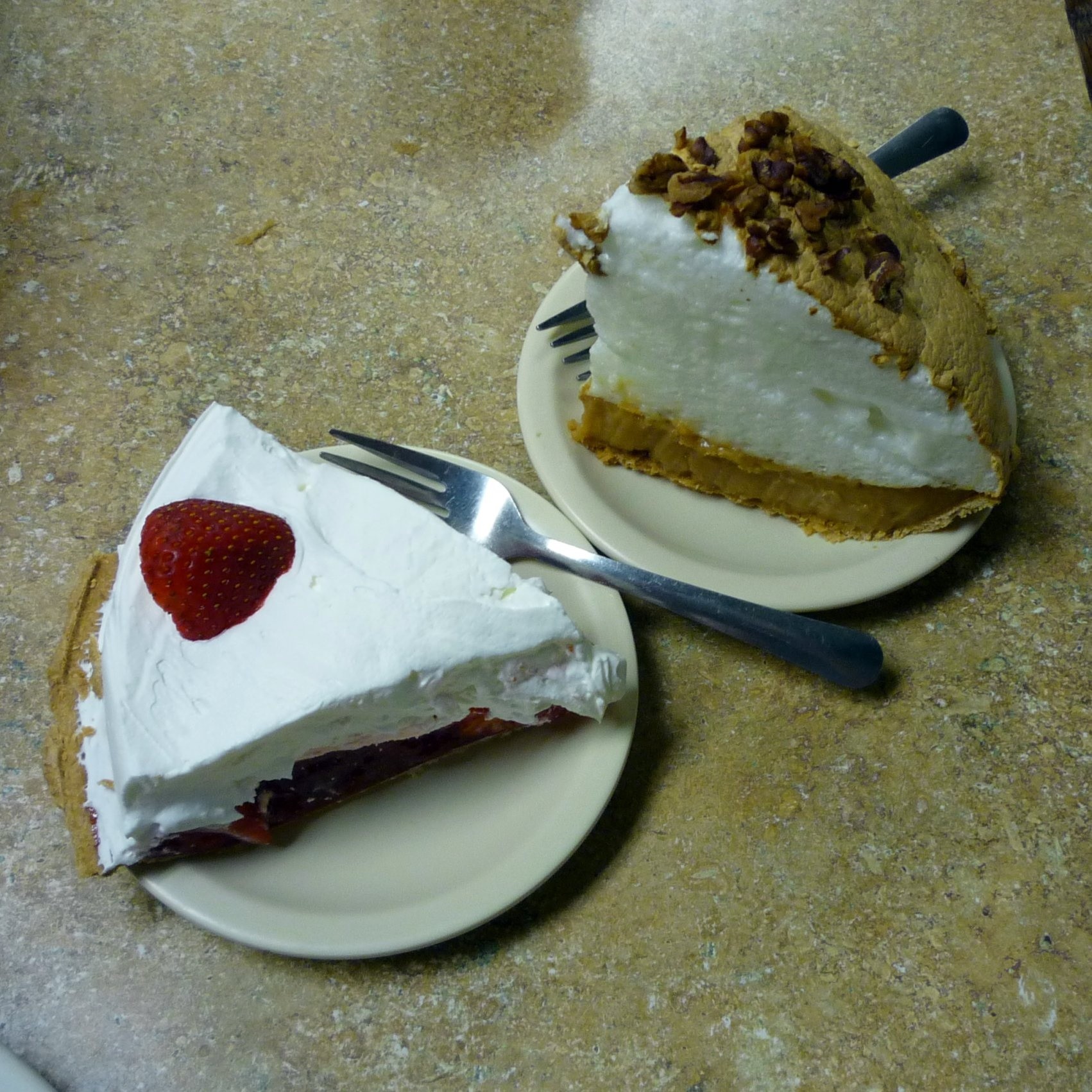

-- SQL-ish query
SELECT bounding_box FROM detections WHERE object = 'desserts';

[48,399,629,868]
[547,103,1018,539]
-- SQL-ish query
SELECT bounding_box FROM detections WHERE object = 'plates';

[78,443,641,962]
[516,259,1018,611]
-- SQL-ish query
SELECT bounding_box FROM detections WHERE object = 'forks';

[532,107,970,382]
[318,428,885,689]
